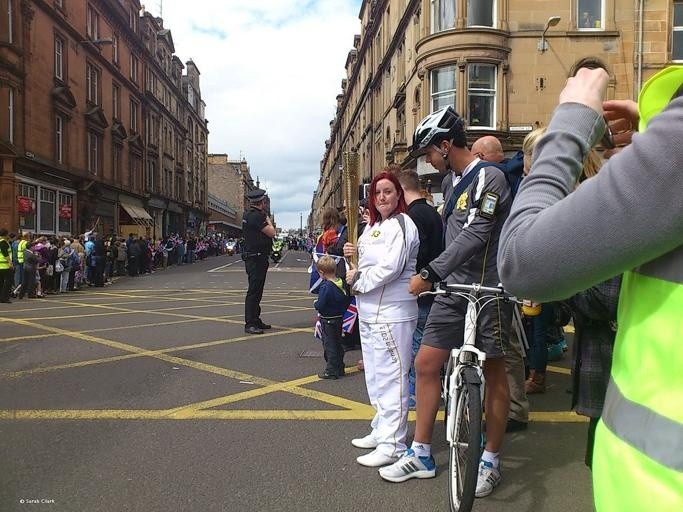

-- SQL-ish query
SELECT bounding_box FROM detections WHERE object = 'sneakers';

[318,371,340,380]
[352,435,380,449]
[474,458,502,498]
[378,448,436,482]
[506,417,528,433]
[356,446,399,468]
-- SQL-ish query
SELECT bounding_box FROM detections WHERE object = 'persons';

[1,126,625,467]
[493,63,682,512]
[376,104,516,497]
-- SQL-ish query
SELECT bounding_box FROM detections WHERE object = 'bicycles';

[415,281,531,511]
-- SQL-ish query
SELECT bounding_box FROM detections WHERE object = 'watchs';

[421,265,433,283]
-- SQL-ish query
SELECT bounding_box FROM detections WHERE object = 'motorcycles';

[224,242,236,256]
[271,240,283,263]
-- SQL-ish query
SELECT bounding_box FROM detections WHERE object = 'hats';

[64,240,71,245]
[247,189,265,199]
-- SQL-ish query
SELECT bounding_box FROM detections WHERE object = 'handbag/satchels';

[55,260,64,272]
[46,264,53,276]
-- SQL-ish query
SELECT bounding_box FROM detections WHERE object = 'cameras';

[601,115,616,149]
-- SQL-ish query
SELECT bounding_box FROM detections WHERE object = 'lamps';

[537,15,562,54]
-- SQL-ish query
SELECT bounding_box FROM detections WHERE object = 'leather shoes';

[258,323,271,329]
[245,326,264,334]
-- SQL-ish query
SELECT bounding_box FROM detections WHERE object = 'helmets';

[407,104,465,158]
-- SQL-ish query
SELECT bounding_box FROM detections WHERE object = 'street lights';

[299,211,302,234]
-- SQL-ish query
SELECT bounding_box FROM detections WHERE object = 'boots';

[525,368,546,394]
